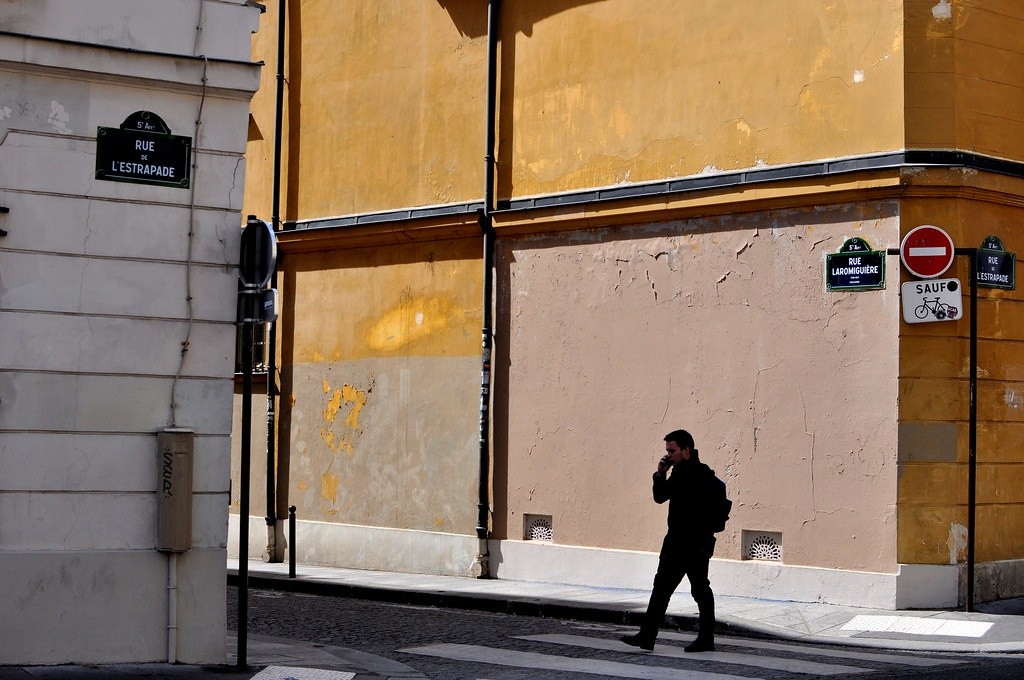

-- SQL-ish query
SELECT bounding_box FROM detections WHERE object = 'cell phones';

[661,456,674,468]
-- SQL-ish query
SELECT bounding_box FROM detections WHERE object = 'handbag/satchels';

[710,469,732,532]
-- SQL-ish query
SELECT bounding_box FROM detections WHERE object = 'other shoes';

[619,633,656,651]
[684,641,715,652]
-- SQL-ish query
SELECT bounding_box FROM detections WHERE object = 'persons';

[616,428,732,653]
